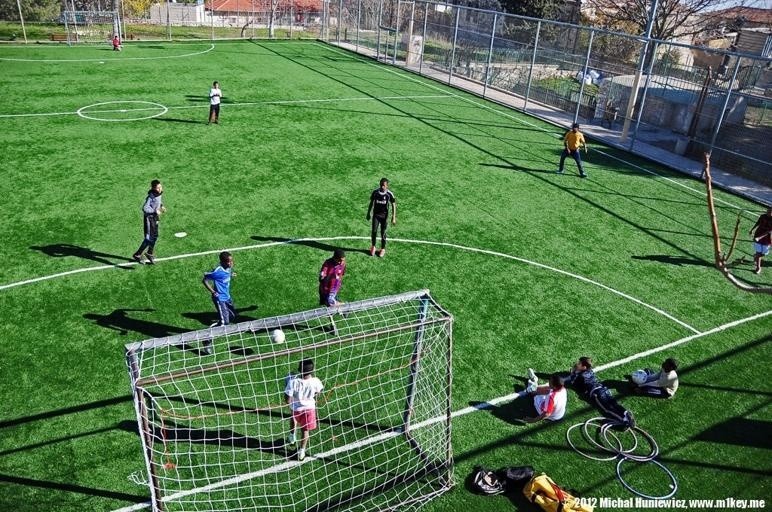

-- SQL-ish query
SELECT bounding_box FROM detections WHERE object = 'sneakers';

[202,341,211,355]
[131,252,146,266]
[527,379,538,393]
[296,448,306,460]
[379,249,386,257]
[143,251,155,264]
[580,173,588,177]
[623,419,629,430]
[369,246,376,255]
[556,170,564,173]
[528,368,539,382]
[287,432,297,447]
[626,410,637,429]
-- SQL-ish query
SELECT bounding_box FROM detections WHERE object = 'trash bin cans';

[674,136,689,156]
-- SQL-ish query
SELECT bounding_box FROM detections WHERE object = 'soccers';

[272,330,285,343]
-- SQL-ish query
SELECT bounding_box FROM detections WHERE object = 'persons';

[365,177,396,257]
[515,368,568,422]
[200,251,239,354]
[567,356,636,431]
[555,123,588,177]
[585,93,618,130]
[748,207,771,275]
[282,359,325,460]
[113,36,121,51]
[318,247,352,336]
[206,82,223,126]
[133,179,168,266]
[632,357,679,400]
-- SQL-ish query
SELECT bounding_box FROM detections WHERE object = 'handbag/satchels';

[524,473,595,512]
[473,470,507,497]
[506,466,535,483]
